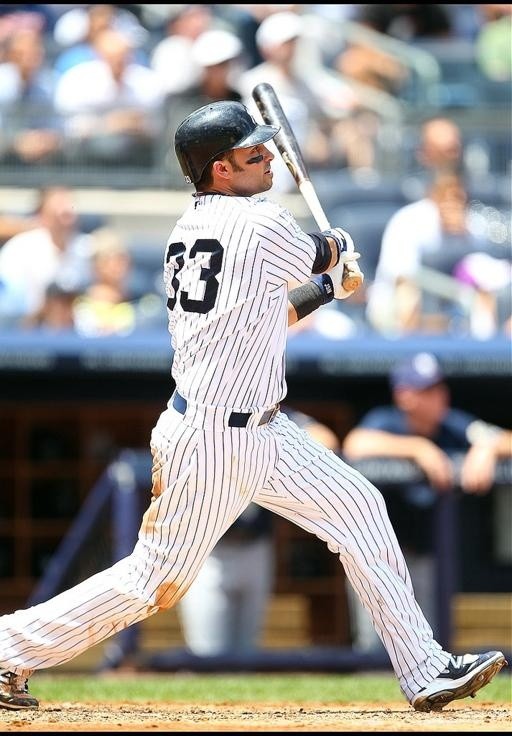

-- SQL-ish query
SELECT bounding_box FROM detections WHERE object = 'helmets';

[174,99,282,184]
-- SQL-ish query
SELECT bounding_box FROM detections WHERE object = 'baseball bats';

[252,82,364,292]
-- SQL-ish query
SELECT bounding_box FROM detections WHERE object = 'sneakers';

[409,650,506,712]
[0,667,40,710]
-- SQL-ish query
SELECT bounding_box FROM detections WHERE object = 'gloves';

[311,251,365,304]
[323,227,356,254]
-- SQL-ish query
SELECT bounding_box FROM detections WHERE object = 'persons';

[173,391,340,656]
[0,166,511,338]
[336,347,511,654]
[0,98,506,717]
[0,2,511,192]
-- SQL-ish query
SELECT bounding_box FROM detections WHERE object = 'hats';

[390,350,450,393]
[46,280,85,298]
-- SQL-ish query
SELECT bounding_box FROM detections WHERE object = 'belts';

[172,389,281,428]
